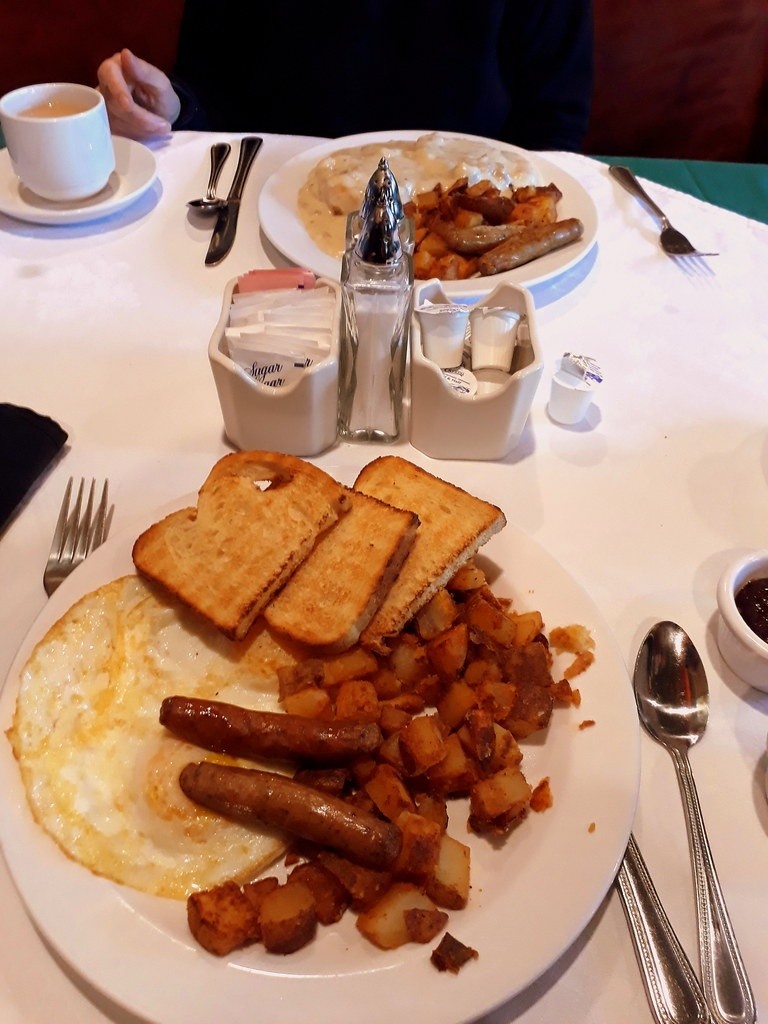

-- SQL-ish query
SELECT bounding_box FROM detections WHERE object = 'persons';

[90,0,595,155]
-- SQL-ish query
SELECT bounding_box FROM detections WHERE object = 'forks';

[607,163,719,261]
[41,474,112,601]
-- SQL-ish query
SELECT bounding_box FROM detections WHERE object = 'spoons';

[185,141,232,215]
[630,618,758,1024]
[614,834,713,1024]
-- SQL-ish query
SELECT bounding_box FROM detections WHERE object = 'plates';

[1,480,641,1024]
[0,133,159,227]
[258,131,598,296]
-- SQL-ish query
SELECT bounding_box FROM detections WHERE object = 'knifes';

[205,136,264,269]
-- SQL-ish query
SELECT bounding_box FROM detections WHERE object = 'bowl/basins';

[717,552,768,693]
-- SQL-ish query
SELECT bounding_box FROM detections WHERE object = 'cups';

[1,82,115,203]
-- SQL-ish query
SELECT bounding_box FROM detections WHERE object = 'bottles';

[344,151,414,277]
[333,189,406,444]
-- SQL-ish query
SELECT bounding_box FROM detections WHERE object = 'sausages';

[159,696,407,867]
[447,218,583,275]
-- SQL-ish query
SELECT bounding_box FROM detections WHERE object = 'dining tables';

[1,128,768,1022]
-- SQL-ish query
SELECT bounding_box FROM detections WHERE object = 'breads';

[128,454,506,650]
